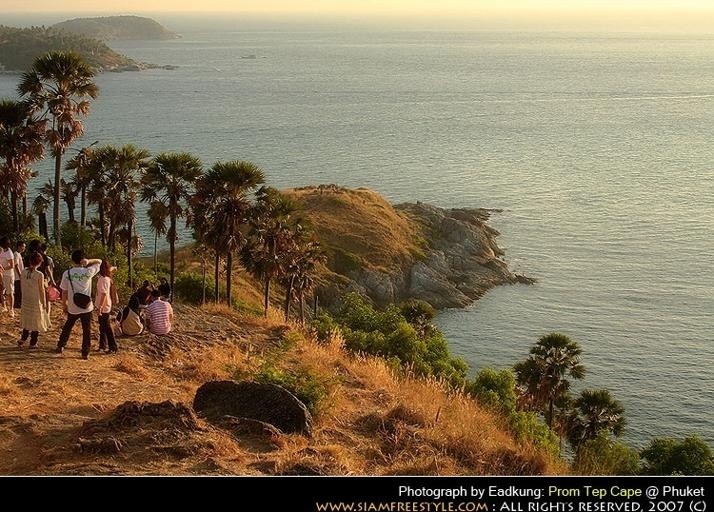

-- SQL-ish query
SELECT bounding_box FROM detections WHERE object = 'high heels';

[95,347,118,354]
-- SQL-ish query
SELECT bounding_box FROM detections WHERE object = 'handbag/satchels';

[114,322,123,337]
[73,293,90,309]
[109,284,119,305]
[46,284,60,302]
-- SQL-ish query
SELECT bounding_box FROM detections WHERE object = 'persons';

[0,237,174,362]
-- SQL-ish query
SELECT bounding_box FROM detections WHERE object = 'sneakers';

[0,307,14,317]
[18,339,88,359]
